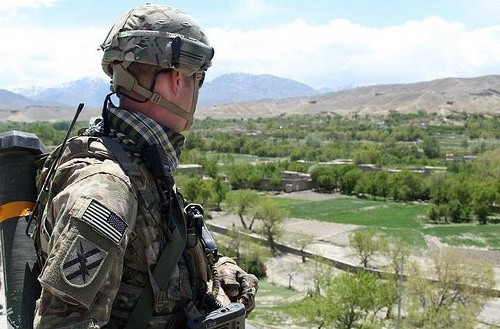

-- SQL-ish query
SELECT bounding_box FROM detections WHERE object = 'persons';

[32,3,260,329]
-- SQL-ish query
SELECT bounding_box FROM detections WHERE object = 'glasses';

[199,73,206,90]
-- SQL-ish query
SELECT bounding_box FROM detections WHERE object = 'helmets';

[100,3,215,73]
